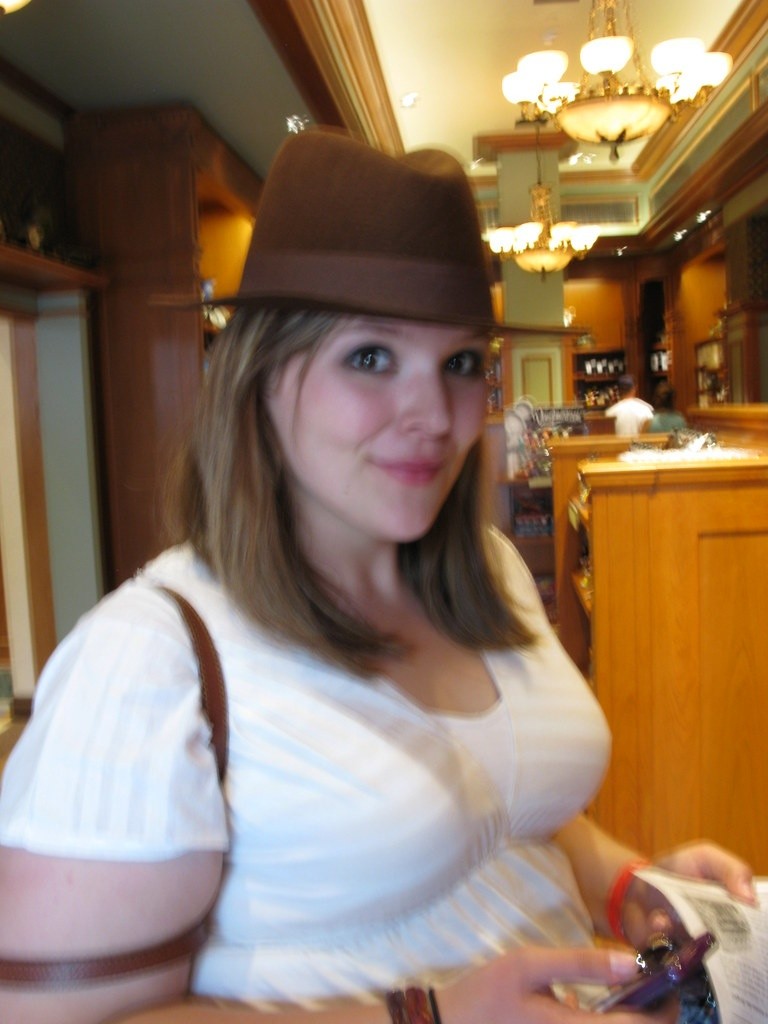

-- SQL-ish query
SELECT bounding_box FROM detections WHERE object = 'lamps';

[501,0,738,164]
[482,119,600,283]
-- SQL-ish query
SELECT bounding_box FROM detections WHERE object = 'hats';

[147,125,590,342]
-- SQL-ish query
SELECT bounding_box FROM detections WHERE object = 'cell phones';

[589,933,716,1013]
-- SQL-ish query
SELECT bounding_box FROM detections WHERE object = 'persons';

[641,381,688,434]
[0,127,759,1024]
[606,375,653,436]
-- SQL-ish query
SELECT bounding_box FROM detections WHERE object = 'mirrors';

[669,160,768,420]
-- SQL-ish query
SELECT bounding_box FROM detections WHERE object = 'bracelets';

[385,985,443,1024]
[605,860,652,944]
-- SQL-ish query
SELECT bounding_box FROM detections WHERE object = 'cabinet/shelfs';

[572,347,627,409]
[542,426,768,886]
